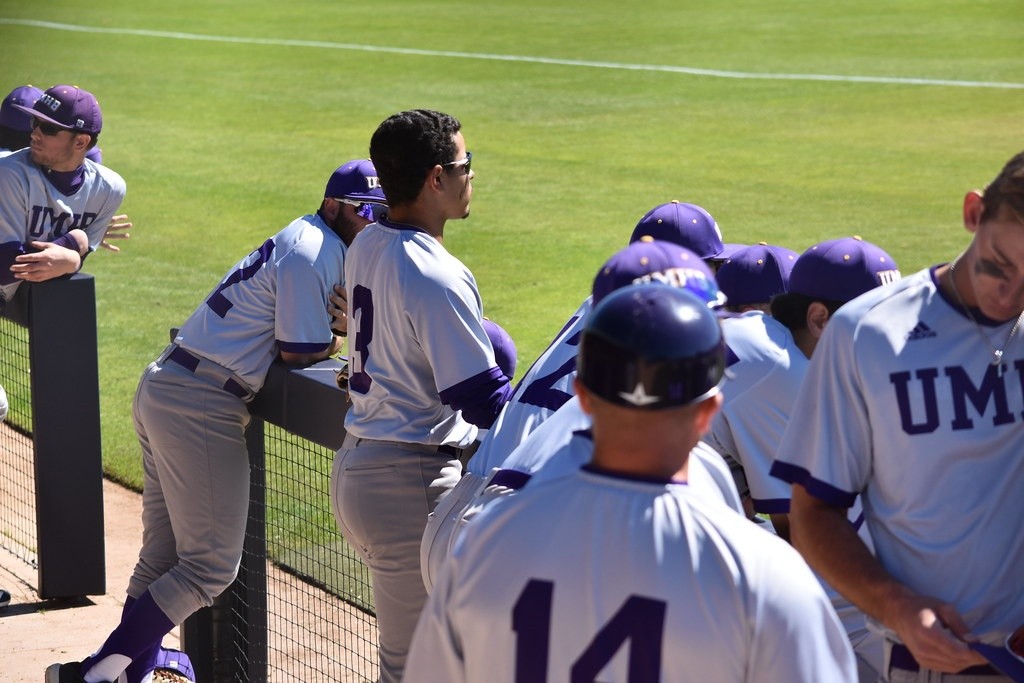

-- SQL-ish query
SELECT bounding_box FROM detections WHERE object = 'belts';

[888,642,1000,675]
[485,469,532,490]
[169,347,255,405]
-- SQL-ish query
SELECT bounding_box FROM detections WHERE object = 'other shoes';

[0,590,11,608]
[45,656,118,683]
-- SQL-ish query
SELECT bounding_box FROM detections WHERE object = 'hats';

[591,237,727,310]
[629,201,751,259]
[787,235,902,304]
[324,159,387,202]
[482,316,516,378]
[714,241,800,306]
[13,85,103,133]
[0,85,44,132]
[968,638,1024,683]
[575,283,741,408]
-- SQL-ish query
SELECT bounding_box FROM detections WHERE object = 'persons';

[399,284,859,683]
[0,84,132,607]
[45,158,389,683]
[330,109,515,683]
[768,150,1024,683]
[420,200,900,597]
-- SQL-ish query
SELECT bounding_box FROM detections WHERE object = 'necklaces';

[948,252,1023,366]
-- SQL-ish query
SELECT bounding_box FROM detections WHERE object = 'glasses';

[30,117,65,136]
[335,198,389,226]
[442,152,472,174]
